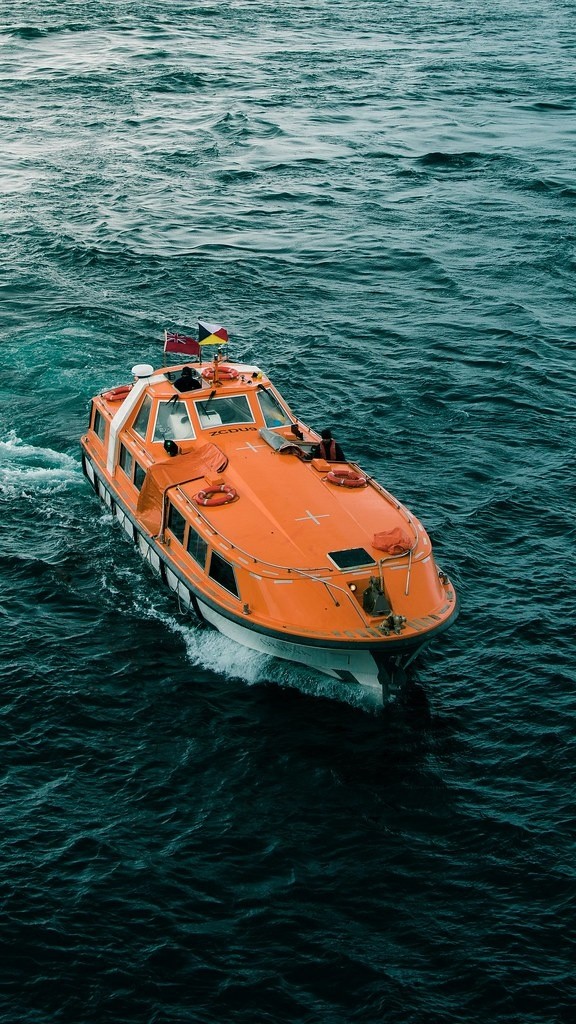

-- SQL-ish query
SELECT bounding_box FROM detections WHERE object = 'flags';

[198,320,228,345]
[164,330,200,357]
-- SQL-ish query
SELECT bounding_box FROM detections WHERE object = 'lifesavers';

[325,469,367,488]
[194,484,237,507]
[105,385,133,402]
[205,366,240,380]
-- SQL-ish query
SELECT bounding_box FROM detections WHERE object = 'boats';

[80,359,461,696]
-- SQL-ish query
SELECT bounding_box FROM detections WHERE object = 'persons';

[173,366,203,392]
[305,429,345,461]
